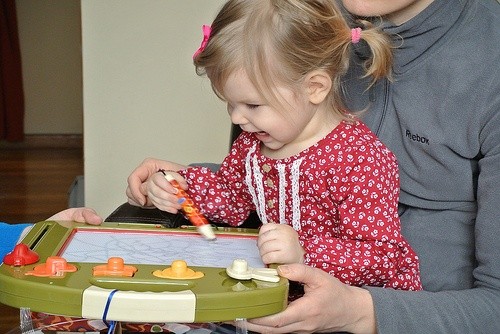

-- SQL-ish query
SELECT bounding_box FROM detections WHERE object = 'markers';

[164,174,216,242]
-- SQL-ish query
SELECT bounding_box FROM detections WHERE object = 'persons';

[147,0,423,305]
[126,0,500,334]
[0,207,102,266]
